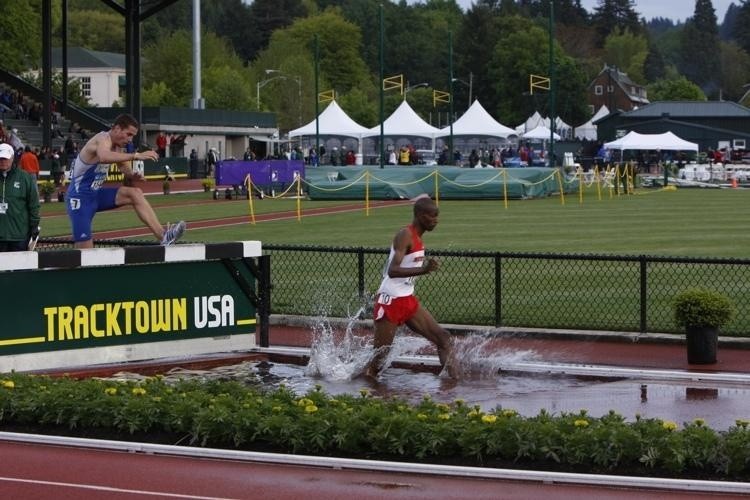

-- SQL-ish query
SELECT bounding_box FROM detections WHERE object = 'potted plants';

[667,286,736,365]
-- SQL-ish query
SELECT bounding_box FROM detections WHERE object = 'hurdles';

[0,240,274,373]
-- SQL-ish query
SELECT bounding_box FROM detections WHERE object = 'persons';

[263,140,549,166]
[367,197,460,382]
[244,149,255,162]
[190,149,197,179]
[1,142,41,250]
[0,81,91,186]
[157,129,167,156]
[169,131,177,142]
[64,112,187,249]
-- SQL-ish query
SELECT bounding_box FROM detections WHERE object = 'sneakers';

[160,220,186,245]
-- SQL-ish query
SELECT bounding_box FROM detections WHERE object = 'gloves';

[28,227,39,241]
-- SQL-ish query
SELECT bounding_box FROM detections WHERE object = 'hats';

[0,143,14,159]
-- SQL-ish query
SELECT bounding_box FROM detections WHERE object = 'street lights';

[403,82,428,100]
[451,71,473,106]
[256,69,302,128]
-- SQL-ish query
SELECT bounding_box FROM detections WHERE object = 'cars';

[415,149,438,166]
[456,153,470,168]
